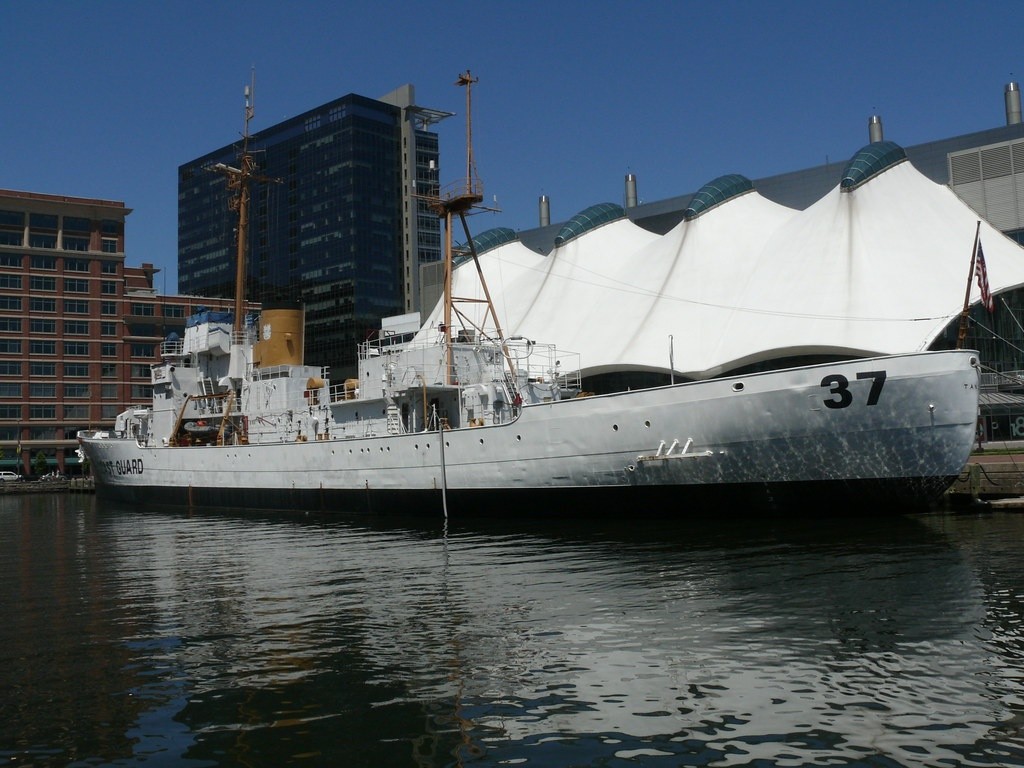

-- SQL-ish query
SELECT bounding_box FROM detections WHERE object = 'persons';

[49,468,61,480]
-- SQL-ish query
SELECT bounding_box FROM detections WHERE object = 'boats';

[71,68,986,529]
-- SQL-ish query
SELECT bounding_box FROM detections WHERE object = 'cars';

[40,473,67,481]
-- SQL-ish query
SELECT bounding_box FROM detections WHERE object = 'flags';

[973,241,995,313]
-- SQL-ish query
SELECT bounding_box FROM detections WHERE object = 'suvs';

[0,471,24,482]
